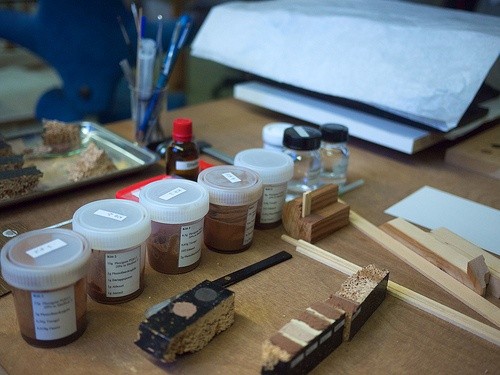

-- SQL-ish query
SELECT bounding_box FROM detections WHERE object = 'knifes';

[145,251,292,320]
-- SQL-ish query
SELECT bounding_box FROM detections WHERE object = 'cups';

[128,85,168,145]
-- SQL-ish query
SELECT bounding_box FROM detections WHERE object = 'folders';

[232,77,490,154]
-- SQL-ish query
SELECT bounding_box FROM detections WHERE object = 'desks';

[0,97,500,375]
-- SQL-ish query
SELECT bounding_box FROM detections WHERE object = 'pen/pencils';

[117,1,195,145]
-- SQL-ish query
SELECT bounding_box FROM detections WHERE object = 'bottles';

[164,118,199,183]
[283,124,350,203]
